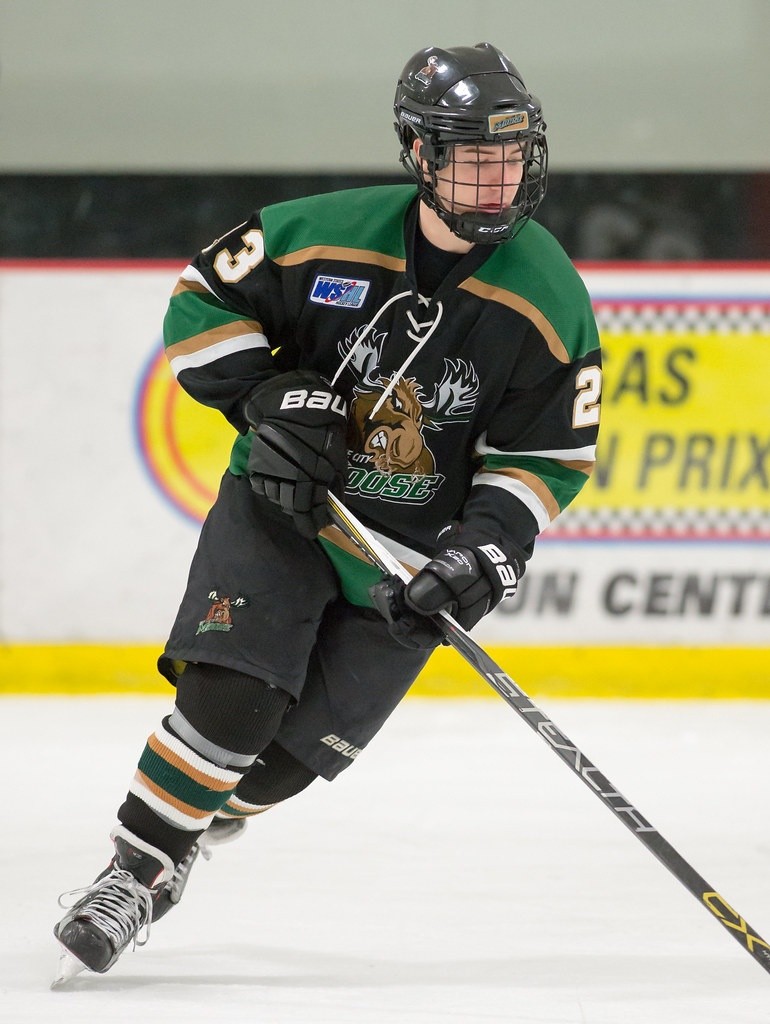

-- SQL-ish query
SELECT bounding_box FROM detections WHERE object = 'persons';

[47,41,605,974]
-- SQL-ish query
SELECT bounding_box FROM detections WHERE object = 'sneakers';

[44,831,211,991]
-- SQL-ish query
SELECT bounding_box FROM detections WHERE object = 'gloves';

[368,474,552,651]
[243,370,348,538]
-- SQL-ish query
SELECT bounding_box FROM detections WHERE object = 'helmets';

[392,43,548,244]
